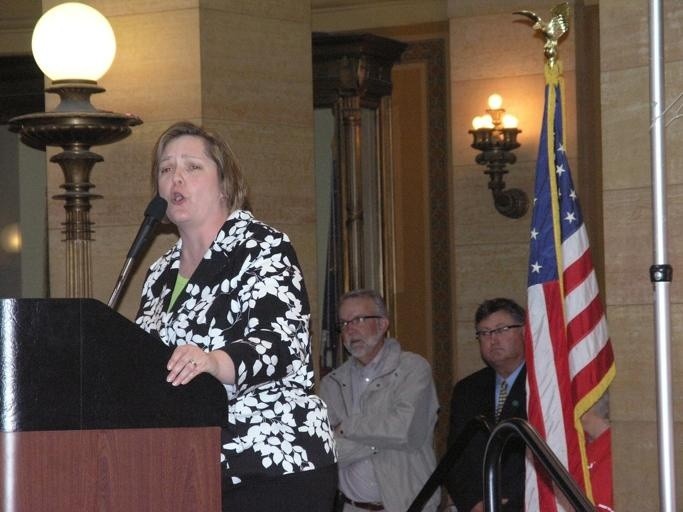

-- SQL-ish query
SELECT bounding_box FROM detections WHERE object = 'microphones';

[106,196,168,311]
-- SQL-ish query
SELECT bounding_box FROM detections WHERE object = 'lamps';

[7,1,143,298]
[468,93,530,218]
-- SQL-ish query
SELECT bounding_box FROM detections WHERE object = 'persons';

[129,118,342,509]
[445,297,531,510]
[320,284,443,511]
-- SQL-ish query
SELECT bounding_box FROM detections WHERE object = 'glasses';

[334,316,383,332]
[476,324,522,339]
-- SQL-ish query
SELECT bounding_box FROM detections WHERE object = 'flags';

[517,57,620,512]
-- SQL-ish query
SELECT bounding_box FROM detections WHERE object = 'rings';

[188,359,197,368]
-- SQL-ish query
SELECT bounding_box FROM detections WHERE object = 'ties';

[496,381,508,423]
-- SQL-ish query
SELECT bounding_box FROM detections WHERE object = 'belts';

[342,494,384,510]
